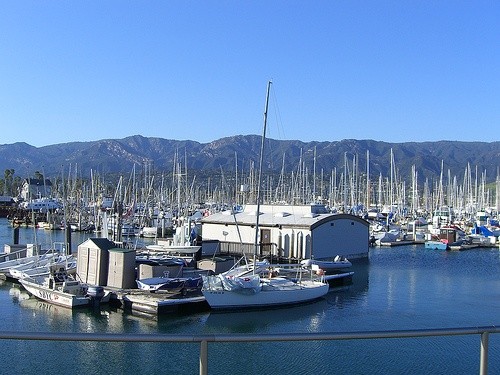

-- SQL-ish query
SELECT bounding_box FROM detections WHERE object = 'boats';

[19,274,113,310]
[8,163,74,278]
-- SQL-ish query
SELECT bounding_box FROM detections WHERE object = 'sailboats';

[77,151,201,240]
[201,78,330,311]
[331,150,500,242]
[0,165,58,279]
[19,165,78,279]
